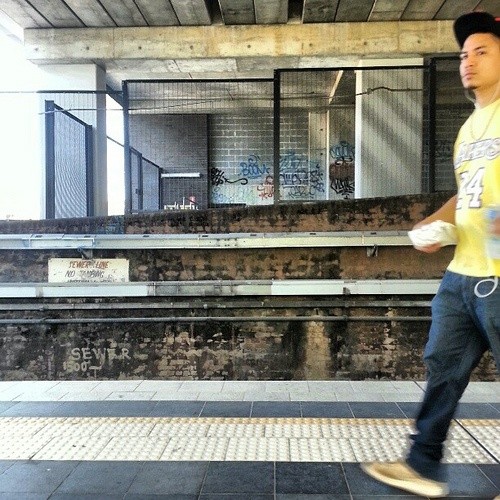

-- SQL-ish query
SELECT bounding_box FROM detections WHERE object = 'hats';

[453,11,499,46]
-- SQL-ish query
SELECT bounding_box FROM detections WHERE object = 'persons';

[358,10,499,499]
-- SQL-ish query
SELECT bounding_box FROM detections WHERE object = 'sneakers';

[365,462,444,497]
